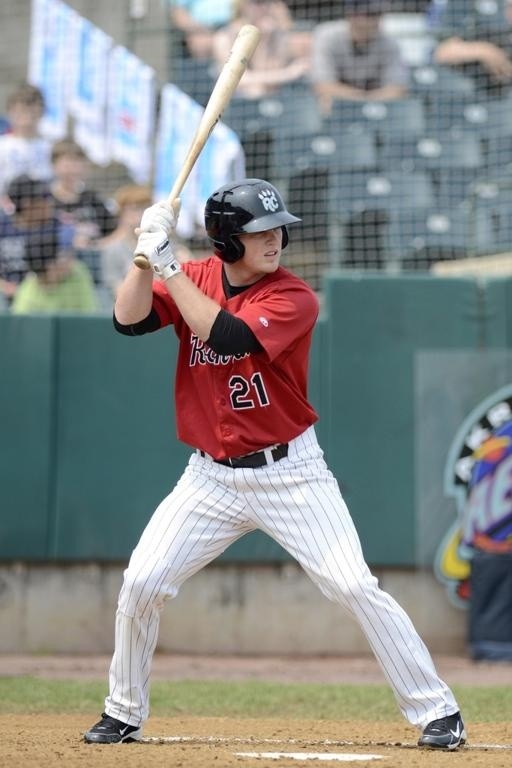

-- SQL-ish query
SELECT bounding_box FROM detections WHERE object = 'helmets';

[204,178,301,264]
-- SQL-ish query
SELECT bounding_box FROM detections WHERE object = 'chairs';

[186,54,511,275]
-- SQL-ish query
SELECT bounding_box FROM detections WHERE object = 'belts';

[201,442,288,469]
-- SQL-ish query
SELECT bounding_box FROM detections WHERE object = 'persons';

[0,83,152,315]
[170,0,512,119]
[81,176,468,753]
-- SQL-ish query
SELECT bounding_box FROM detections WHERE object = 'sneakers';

[417,712,467,751]
[84,713,143,745]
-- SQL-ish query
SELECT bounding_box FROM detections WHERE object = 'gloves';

[134,222,182,283]
[139,197,182,240]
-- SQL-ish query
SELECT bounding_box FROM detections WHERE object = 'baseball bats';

[133,22,262,271]
